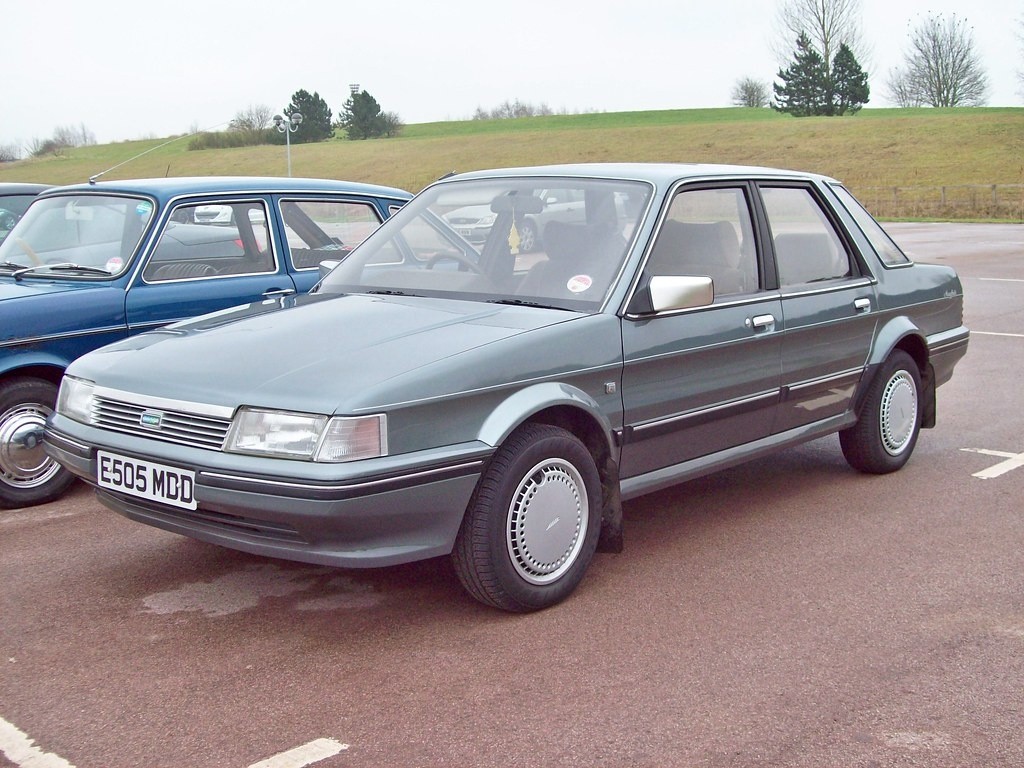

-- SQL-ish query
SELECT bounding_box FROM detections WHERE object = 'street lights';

[272,111,304,177]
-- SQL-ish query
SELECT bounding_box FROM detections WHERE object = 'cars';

[439,185,647,254]
[193,204,266,224]
[170,207,194,224]
[0,181,264,267]
[0,174,485,509]
[41,161,971,613]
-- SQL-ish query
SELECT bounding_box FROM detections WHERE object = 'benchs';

[262,247,351,269]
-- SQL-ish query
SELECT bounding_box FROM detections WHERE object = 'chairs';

[773,232,839,284]
[646,219,742,295]
[515,221,614,302]
[151,264,216,280]
